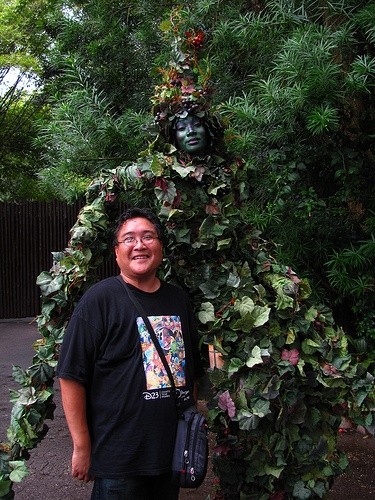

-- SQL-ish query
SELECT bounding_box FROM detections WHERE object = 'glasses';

[118,235,159,247]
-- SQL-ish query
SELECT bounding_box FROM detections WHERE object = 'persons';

[169,113,210,156]
[55,208,202,500]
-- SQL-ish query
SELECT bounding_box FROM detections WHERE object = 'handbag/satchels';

[168,406,209,490]
